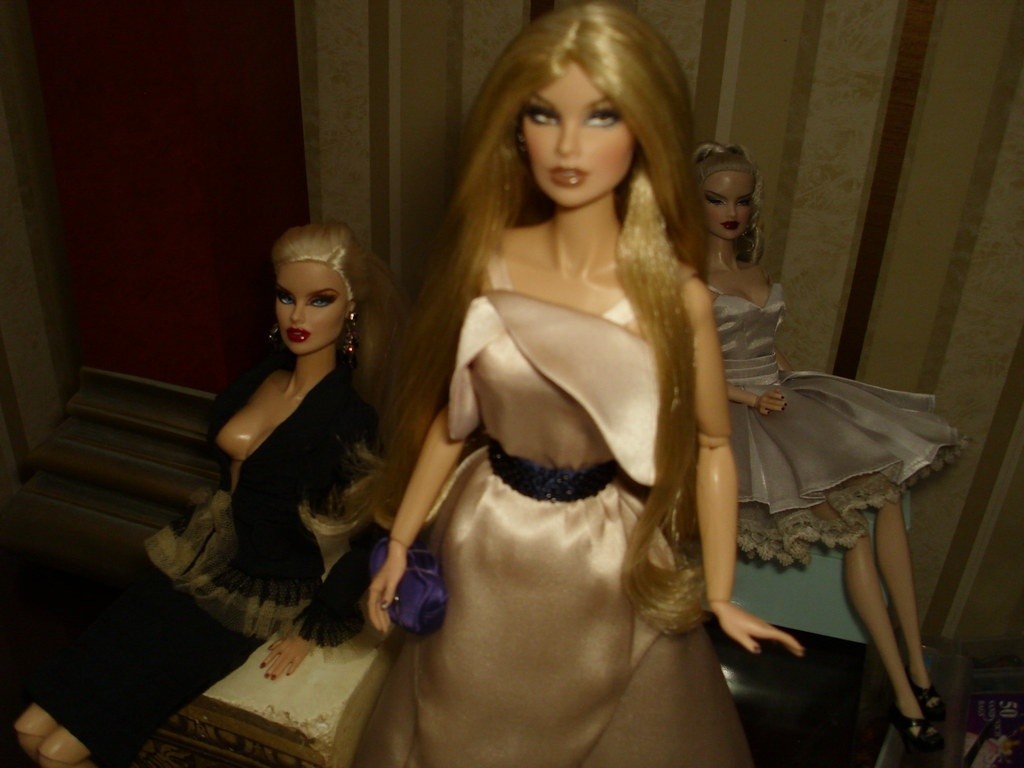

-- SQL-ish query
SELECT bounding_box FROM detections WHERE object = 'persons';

[689,142,979,756]
[12,218,386,768]
[367,1,806,768]
